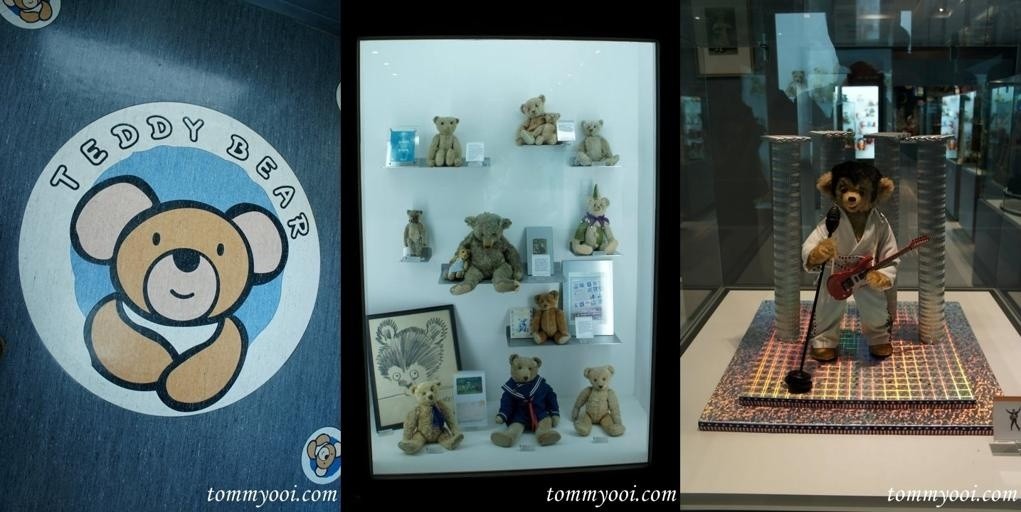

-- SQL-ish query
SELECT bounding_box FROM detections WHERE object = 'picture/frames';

[690,1,756,79]
[562,260,614,337]
[364,304,462,432]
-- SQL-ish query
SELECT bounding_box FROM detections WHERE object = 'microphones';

[824,205,841,233]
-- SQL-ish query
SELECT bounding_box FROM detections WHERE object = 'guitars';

[826,235,929,299]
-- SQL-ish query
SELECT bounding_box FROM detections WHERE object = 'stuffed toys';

[570,183,619,255]
[783,57,854,107]
[442,245,471,282]
[573,118,621,166]
[529,289,571,344]
[490,354,562,448]
[450,212,524,295]
[397,379,465,454]
[801,160,904,361]
[572,364,627,436]
[403,209,427,257]
[428,115,464,167]
[515,95,561,146]
